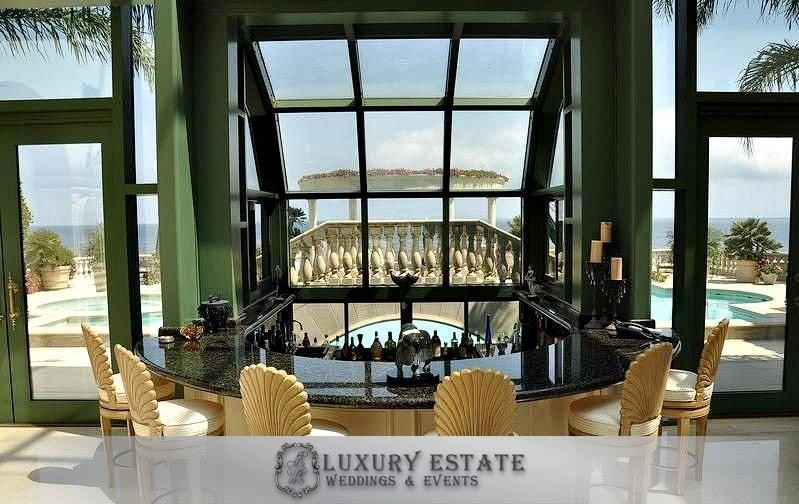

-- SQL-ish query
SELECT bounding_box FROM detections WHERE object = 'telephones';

[616,322,656,341]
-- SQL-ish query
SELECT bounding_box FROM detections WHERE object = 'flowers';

[25,269,42,290]
[760,259,783,274]
[302,168,503,178]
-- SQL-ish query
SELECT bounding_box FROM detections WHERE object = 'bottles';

[256,313,548,361]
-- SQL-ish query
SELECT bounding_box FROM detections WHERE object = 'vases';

[761,271,778,285]
[26,287,34,294]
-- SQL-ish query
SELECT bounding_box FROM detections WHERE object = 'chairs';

[81,323,176,488]
[238,365,350,437]
[566,342,674,504]
[113,343,225,504]
[652,318,729,496]
[422,367,521,436]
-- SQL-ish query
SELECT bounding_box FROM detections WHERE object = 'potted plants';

[38,247,77,290]
[721,217,784,283]
[88,232,108,292]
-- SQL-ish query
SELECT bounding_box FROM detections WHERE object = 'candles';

[600,221,612,243]
[590,240,603,264]
[610,257,623,280]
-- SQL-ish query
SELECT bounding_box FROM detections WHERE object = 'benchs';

[139,260,152,285]
[657,255,674,272]
